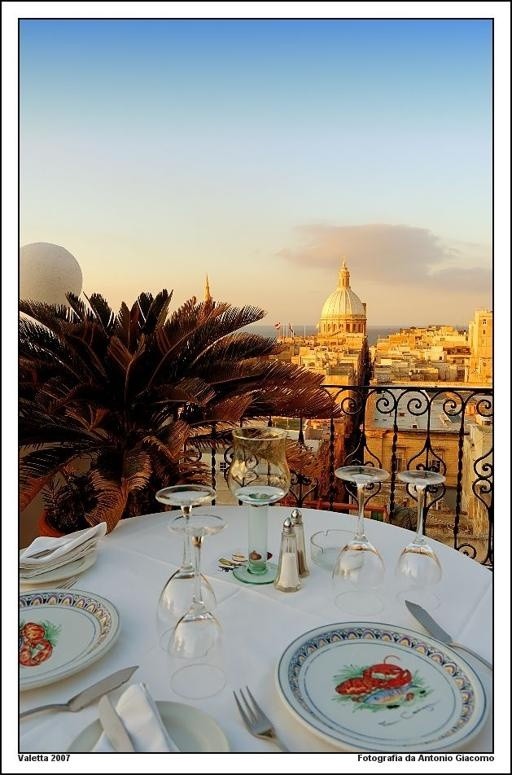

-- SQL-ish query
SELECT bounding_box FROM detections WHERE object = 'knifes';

[21,666,140,728]
[96,699,134,753]
[404,600,493,673]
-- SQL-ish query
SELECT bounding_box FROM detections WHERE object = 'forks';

[232,685,289,752]
[59,573,82,591]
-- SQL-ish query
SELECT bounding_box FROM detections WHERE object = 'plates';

[66,700,228,753]
[20,543,98,586]
[19,587,125,700]
[273,619,486,753]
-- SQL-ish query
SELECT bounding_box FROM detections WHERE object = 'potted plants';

[18,289,346,538]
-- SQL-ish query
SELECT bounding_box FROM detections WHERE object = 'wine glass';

[393,467,444,589]
[151,484,230,699]
[228,427,289,586]
[332,463,390,589]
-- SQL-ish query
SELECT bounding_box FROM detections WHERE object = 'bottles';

[275,509,309,595]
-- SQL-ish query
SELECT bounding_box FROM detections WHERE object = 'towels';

[94,684,179,752]
[20,522,110,577]
